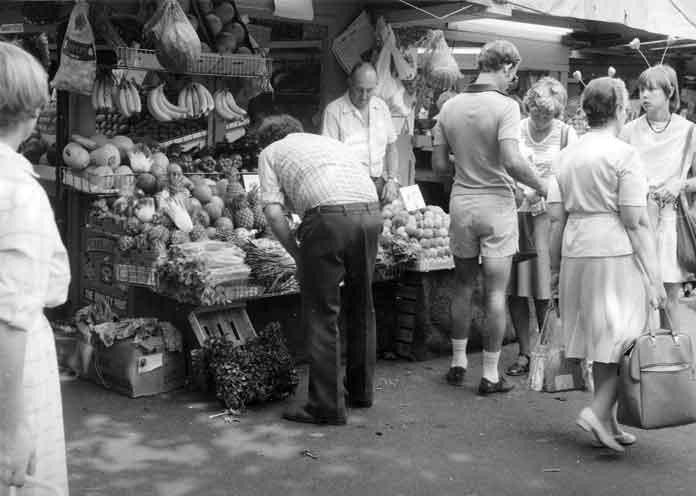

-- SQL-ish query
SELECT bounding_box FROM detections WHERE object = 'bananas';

[92,79,247,120]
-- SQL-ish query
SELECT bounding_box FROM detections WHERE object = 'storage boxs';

[96,335,184,398]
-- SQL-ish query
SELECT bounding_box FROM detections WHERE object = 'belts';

[306,205,380,214]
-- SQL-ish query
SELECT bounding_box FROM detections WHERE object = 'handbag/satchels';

[677,200,696,275]
[620,305,696,430]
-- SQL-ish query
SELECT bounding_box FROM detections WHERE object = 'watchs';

[684,181,689,192]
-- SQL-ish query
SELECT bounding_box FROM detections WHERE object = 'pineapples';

[119,184,265,252]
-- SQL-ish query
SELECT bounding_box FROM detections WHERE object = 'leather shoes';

[506,352,530,375]
[344,396,374,408]
[576,406,624,454]
[281,405,347,425]
[478,376,514,396]
[447,366,465,385]
[591,431,637,447]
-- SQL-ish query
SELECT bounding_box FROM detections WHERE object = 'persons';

[256,115,385,426]
[318,61,400,210]
[617,63,694,334]
[504,76,579,376]
[0,41,71,496]
[548,77,669,451]
[433,42,547,396]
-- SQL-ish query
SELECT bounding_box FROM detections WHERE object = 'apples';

[382,199,452,257]
